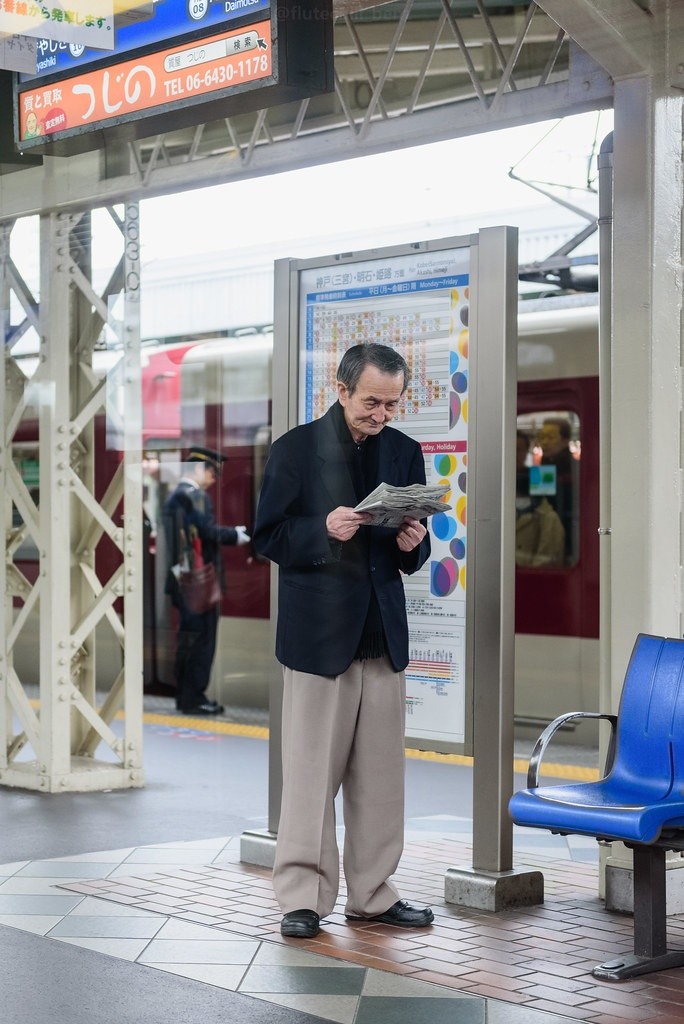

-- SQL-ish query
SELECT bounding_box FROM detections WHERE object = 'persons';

[537,419,581,550]
[515,428,531,465]
[516,465,567,569]
[251,342,431,938]
[164,444,251,715]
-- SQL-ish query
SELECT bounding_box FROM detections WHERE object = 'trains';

[12,250,599,740]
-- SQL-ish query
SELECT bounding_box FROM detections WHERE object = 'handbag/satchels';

[178,561,222,613]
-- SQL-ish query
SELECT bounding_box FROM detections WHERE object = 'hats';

[186,446,228,475]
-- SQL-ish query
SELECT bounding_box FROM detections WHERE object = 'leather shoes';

[177,699,224,715]
[345,899,434,928]
[281,910,320,937]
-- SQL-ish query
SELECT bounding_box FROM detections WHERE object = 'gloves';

[234,525,251,546]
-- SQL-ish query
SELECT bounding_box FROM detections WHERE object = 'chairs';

[507,633,684,982]
